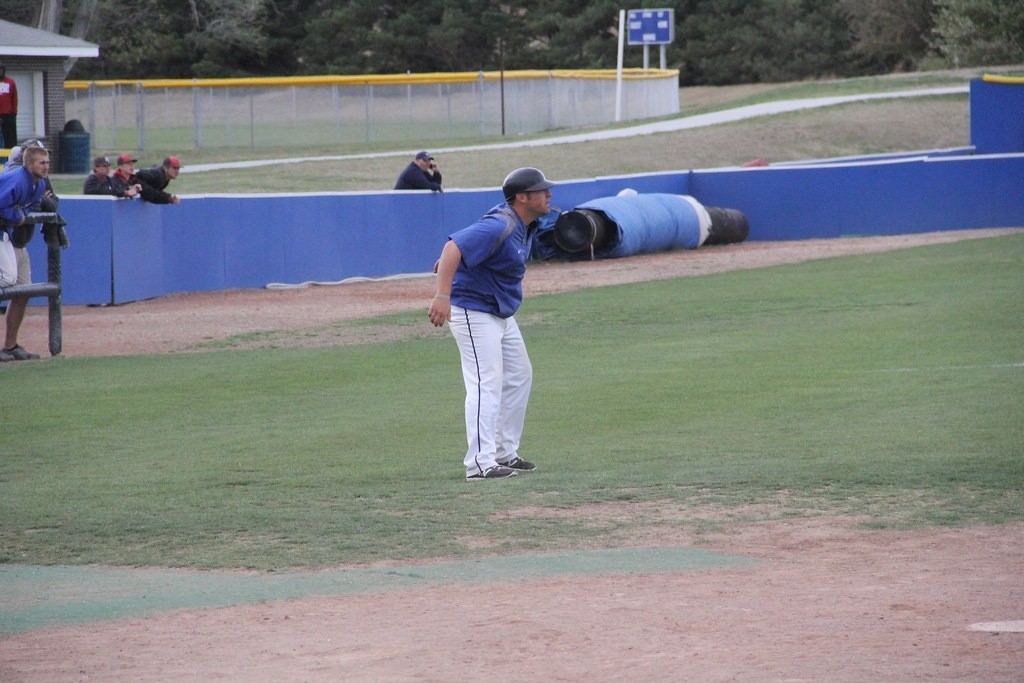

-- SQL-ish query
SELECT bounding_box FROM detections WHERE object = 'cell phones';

[429,164,432,168]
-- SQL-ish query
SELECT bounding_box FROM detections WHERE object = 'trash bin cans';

[59,131,90,175]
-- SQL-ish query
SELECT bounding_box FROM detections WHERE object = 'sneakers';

[467,465,518,481]
[0,342,40,362]
[499,458,536,471]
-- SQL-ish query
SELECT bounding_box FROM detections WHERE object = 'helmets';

[502,167,556,200]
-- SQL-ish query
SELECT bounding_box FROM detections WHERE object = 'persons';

[0,66,18,148]
[0,146,51,362]
[2,139,58,209]
[84,156,118,197]
[428,167,555,482]
[135,157,182,190]
[394,151,442,190]
[111,154,179,204]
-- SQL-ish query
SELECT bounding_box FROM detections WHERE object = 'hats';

[162,156,181,167]
[22,139,44,151]
[93,156,113,166]
[415,151,434,161]
[117,153,136,164]
